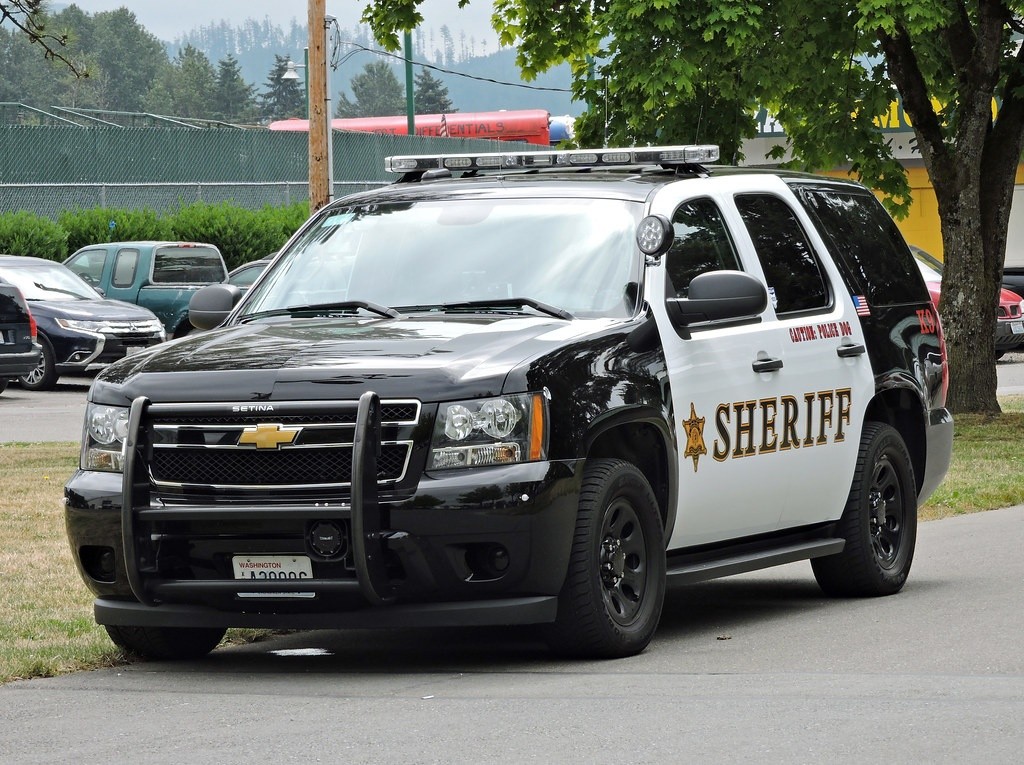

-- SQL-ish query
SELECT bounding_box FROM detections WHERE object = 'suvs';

[61,143,955,659]
[0,277,43,394]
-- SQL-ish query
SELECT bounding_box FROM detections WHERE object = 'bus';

[266,110,581,150]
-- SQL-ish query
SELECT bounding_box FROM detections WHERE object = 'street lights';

[281,46,309,120]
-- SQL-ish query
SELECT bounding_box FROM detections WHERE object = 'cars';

[0,254,167,391]
[907,244,1024,362]
[228,251,279,297]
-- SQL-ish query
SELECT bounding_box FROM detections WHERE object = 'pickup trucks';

[61,241,253,341]
